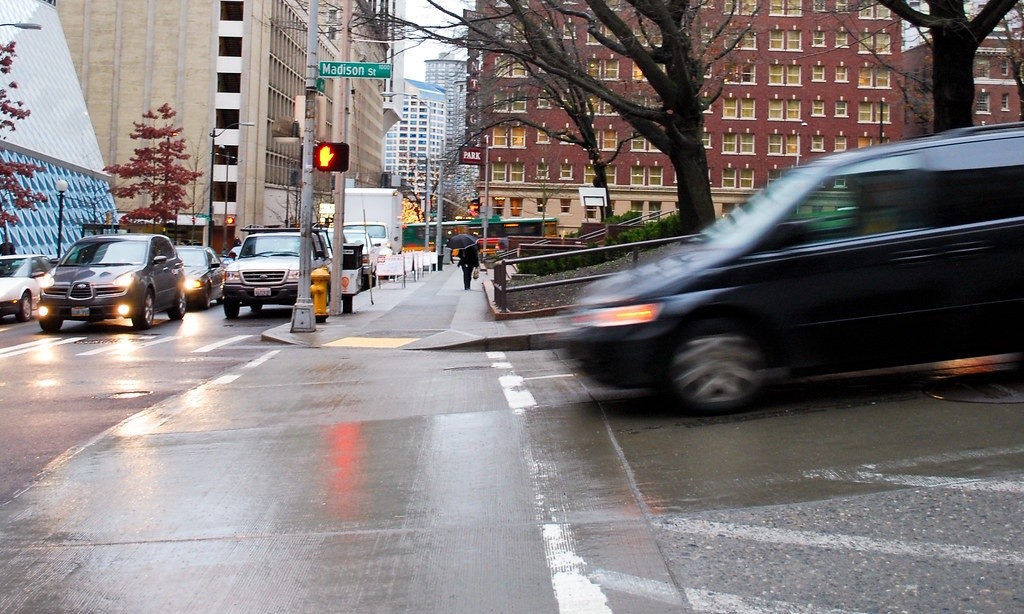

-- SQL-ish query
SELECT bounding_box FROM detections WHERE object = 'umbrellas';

[449,234,479,250]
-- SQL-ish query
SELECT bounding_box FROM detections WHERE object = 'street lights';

[55,179,68,259]
[208,122,255,248]
[381,91,431,252]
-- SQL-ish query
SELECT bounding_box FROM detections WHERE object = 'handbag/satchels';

[473,267,479,279]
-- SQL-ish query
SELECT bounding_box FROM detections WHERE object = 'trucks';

[343,186,404,262]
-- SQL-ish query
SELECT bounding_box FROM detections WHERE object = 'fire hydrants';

[310,263,332,323]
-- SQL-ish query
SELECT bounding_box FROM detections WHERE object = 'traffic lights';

[314,141,349,173]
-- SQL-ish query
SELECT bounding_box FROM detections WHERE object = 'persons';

[0,236,16,267]
[457,247,479,290]
[451,250,454,264]
[234,236,241,245]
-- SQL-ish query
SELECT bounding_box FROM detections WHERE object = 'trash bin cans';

[432,255,444,271]
[341,243,364,295]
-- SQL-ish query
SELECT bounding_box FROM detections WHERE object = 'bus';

[402,217,559,264]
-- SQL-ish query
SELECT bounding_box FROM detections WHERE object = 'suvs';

[221,224,332,320]
[36,232,188,333]
[558,121,1024,418]
[322,228,382,291]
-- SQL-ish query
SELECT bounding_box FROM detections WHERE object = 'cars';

[221,245,243,266]
[0,254,63,323]
[173,245,226,311]
